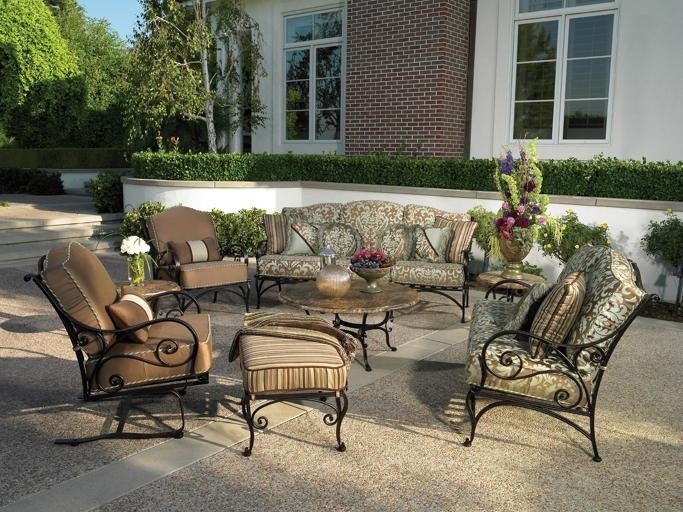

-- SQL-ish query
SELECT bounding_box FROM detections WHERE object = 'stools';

[235,313,348,456]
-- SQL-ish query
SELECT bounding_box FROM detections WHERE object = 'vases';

[128,254,144,286]
[497,232,530,280]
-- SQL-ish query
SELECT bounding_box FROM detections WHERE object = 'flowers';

[491,132,550,251]
[95,234,169,285]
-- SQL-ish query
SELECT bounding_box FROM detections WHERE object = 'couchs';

[462,243,661,462]
[255,199,473,322]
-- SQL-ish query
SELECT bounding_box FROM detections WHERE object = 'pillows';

[167,236,222,264]
[108,284,154,344]
[503,272,587,360]
[261,213,478,263]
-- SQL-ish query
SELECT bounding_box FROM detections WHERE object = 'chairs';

[139,206,251,314]
[23,241,213,447]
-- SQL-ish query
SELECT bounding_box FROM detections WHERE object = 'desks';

[116,279,183,319]
[479,271,543,303]
